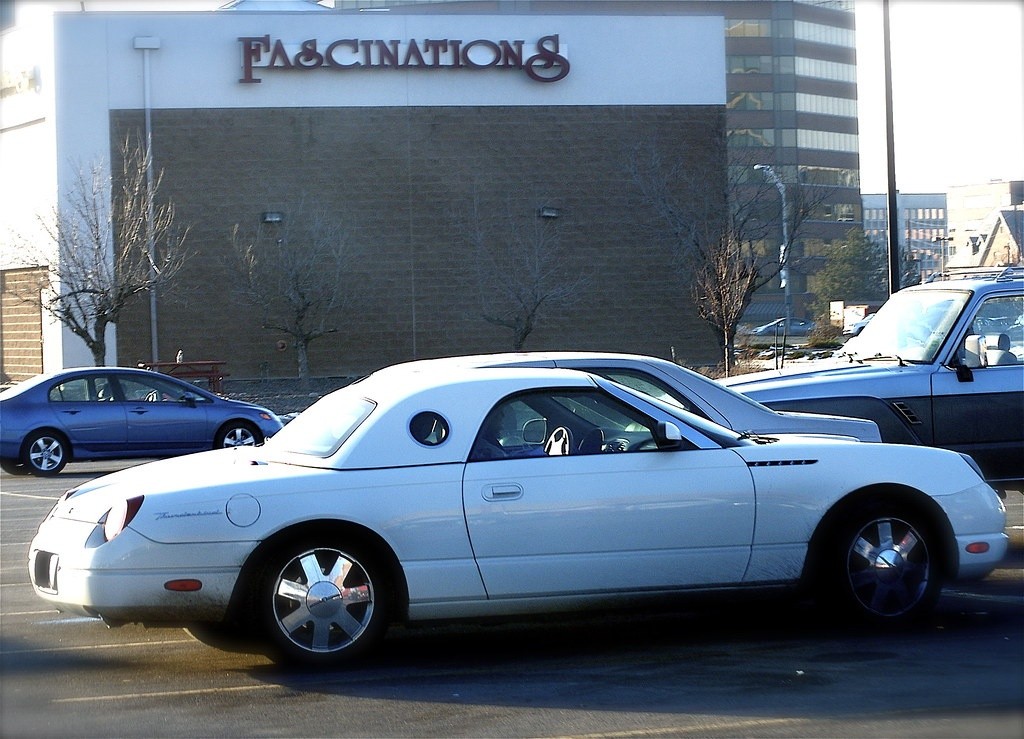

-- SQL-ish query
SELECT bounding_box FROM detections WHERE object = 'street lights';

[1004,246,1010,267]
[754,163,791,335]
[932,236,954,281]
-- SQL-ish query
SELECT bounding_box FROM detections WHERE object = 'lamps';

[262,212,283,222]
[538,206,560,218]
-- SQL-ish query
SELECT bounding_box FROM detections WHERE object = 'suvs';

[625,267,1024,493]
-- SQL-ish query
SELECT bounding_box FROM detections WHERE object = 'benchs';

[146,361,226,392]
[172,370,231,377]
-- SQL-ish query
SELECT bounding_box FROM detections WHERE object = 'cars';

[28,367,1010,669]
[852,313,875,336]
[750,317,816,336]
[401,351,883,458]
[0,367,285,478]
[916,297,1013,335]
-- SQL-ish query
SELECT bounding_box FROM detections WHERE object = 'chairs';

[985,332,1017,366]
[98,383,127,402]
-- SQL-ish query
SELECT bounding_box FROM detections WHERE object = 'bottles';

[176,349,184,364]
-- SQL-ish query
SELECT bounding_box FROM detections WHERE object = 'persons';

[482,409,505,453]
